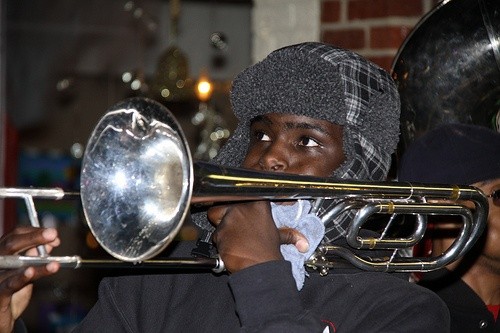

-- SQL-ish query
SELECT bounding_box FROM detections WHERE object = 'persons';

[399,122,500,333]
[0,42,452,333]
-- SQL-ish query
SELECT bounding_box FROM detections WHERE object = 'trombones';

[0,95,488,276]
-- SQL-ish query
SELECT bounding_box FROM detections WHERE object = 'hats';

[190,41,401,247]
